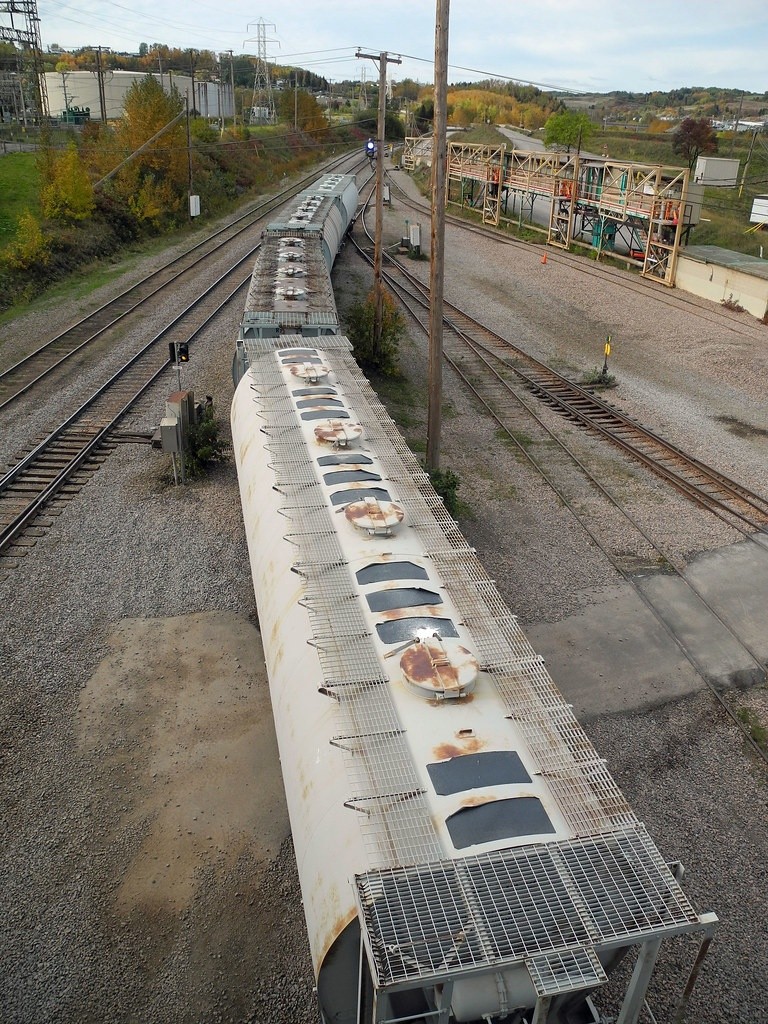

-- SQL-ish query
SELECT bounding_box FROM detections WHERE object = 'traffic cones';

[540,252,547,264]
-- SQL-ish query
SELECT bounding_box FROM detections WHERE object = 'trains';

[231,172,720,1024]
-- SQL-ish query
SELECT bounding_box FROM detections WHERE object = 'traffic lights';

[179,342,189,362]
[365,139,374,158]
